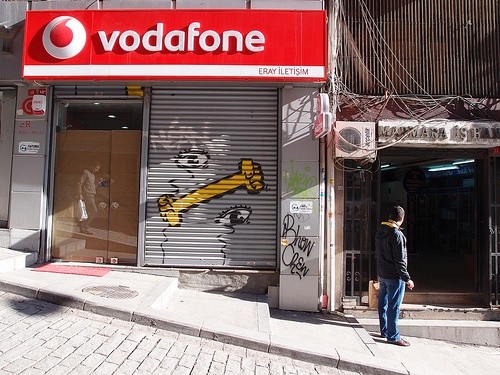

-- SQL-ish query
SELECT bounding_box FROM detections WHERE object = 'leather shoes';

[392,337,410,346]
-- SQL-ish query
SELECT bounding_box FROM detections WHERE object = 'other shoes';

[80,229,93,235]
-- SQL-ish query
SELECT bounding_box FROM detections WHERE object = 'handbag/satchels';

[77,199,88,222]
[369,280,380,309]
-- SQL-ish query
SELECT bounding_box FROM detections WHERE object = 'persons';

[375,205,414,346]
[77,160,101,235]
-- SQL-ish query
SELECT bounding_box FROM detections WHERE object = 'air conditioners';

[336,120,377,160]
